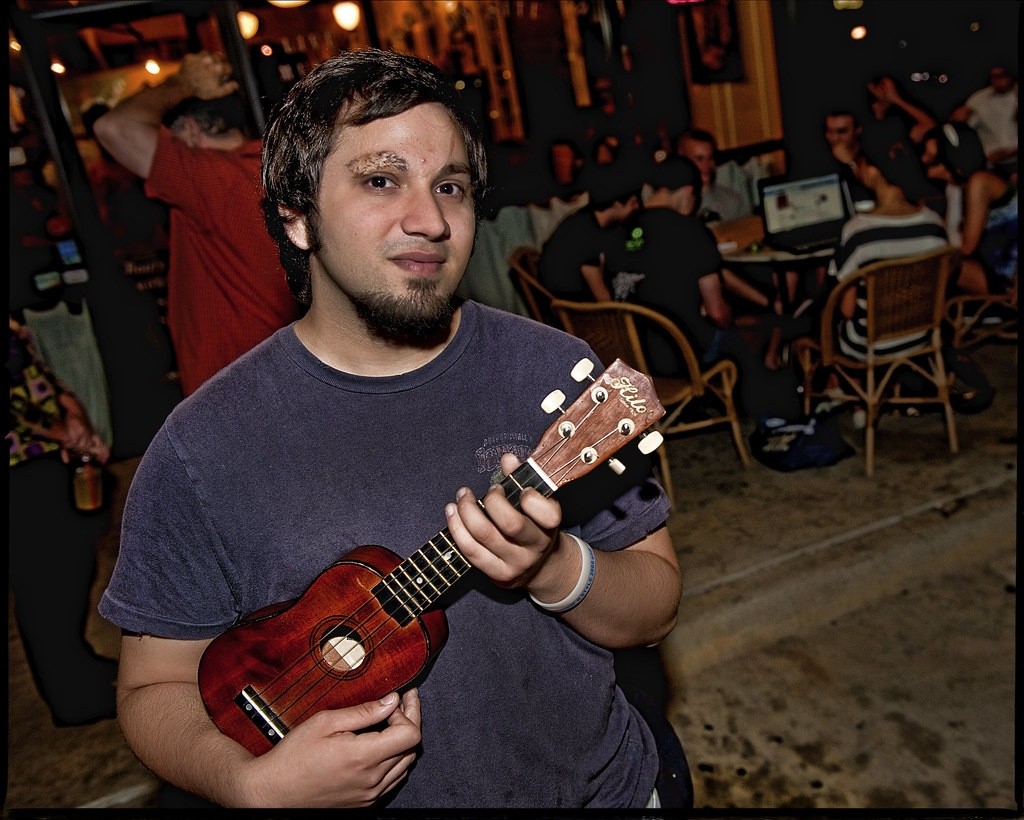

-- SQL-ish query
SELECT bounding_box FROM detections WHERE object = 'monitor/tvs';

[757,166,846,244]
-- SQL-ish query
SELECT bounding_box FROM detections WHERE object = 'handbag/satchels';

[748,399,866,470]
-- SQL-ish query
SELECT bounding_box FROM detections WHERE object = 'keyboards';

[785,236,841,254]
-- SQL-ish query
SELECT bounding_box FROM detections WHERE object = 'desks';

[724,245,836,405]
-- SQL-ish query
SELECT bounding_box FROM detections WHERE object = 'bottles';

[76,456,101,509]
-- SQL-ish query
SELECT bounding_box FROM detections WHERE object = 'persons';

[1,50,1017,726]
[99,51,695,810]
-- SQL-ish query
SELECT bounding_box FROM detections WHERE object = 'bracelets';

[529,532,596,614]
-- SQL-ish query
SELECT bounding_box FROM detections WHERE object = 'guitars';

[196,355,669,759]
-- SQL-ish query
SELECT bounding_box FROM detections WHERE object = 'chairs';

[509,246,1020,513]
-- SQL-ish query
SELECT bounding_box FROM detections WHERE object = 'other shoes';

[94,653,119,685]
[51,685,117,727]
